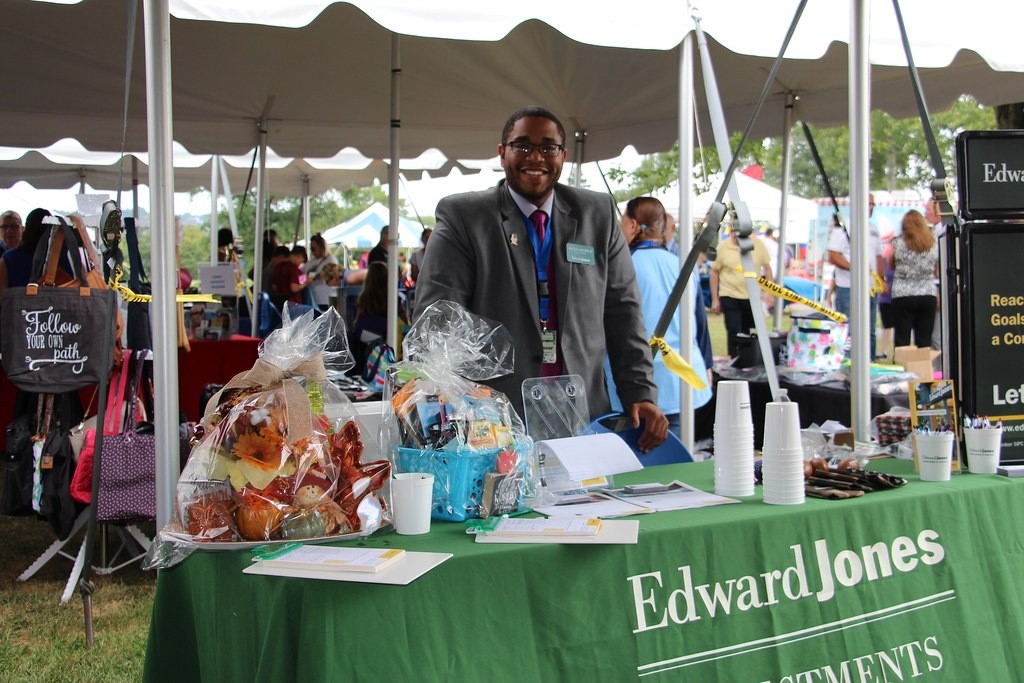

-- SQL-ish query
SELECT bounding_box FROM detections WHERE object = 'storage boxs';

[395,444,537,522]
[896,345,919,369]
[908,351,942,382]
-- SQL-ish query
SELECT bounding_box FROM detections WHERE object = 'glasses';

[510,139,568,157]
[869,201,876,206]
[0,224,21,229]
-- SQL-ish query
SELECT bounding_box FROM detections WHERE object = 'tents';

[0,0,1024,541]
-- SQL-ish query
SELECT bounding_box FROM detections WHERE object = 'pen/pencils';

[913,425,949,436]
[963,413,991,429]
[500,509,534,518]
[996,418,1003,428]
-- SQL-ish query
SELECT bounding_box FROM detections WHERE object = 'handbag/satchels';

[365,340,398,392]
[731,333,787,368]
[4,350,194,541]
[786,314,849,371]
[0,208,125,393]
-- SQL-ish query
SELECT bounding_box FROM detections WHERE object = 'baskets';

[397,433,533,522]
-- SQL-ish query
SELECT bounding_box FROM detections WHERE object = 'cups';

[762,402,805,505]
[392,473,434,535]
[916,431,954,481]
[964,427,1002,474]
[428,419,469,451]
[713,380,755,496]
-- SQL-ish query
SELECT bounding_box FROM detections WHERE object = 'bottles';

[774,389,792,402]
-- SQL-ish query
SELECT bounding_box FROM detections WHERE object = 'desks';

[142,454,1024,683]
[711,364,942,435]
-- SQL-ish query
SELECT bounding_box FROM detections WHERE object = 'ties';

[529,209,562,389]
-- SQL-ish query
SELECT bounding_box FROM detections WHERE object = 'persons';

[0,208,53,289]
[217,104,952,455]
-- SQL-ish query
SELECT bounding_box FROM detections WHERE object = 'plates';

[161,518,389,550]
